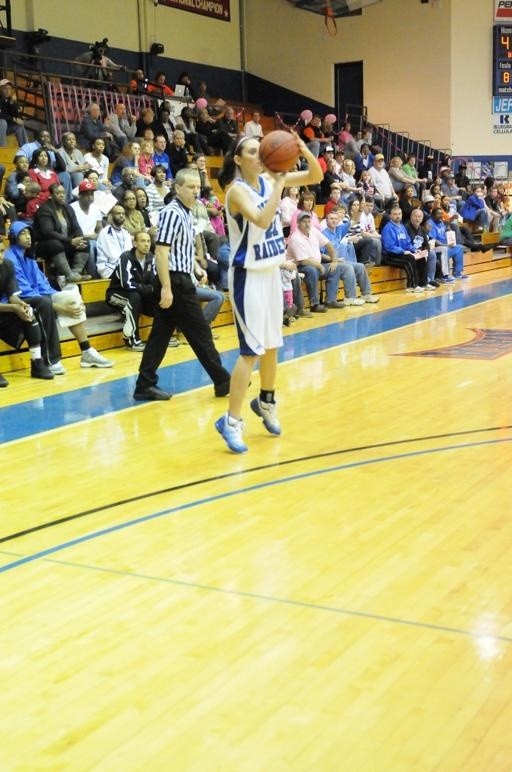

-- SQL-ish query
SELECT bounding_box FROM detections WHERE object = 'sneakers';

[0,374,7,386]
[133,343,146,351]
[437,275,454,282]
[311,304,326,312]
[133,385,171,399]
[30,358,54,379]
[362,294,380,302]
[214,380,251,397]
[251,395,281,435]
[424,284,435,290]
[406,286,424,292]
[344,297,364,305]
[295,311,312,317]
[49,362,64,374]
[327,302,346,308]
[454,272,467,278]
[215,412,248,453]
[80,346,113,367]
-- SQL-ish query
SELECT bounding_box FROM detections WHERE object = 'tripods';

[82,56,106,87]
[20,46,50,120]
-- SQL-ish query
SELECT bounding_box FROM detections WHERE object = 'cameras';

[26,29,53,45]
[89,42,108,57]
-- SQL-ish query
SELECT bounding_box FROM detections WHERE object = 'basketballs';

[259,130,299,172]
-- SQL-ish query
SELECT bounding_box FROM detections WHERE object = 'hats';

[297,212,310,219]
[325,146,333,152]
[440,166,450,172]
[375,153,384,160]
[1,79,10,87]
[79,181,96,192]
[423,195,433,203]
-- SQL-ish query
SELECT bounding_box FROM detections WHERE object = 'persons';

[1,79,510,390]
[131,166,253,401]
[74,43,122,79]
[131,70,174,96]
[213,130,325,456]
[177,72,208,98]
